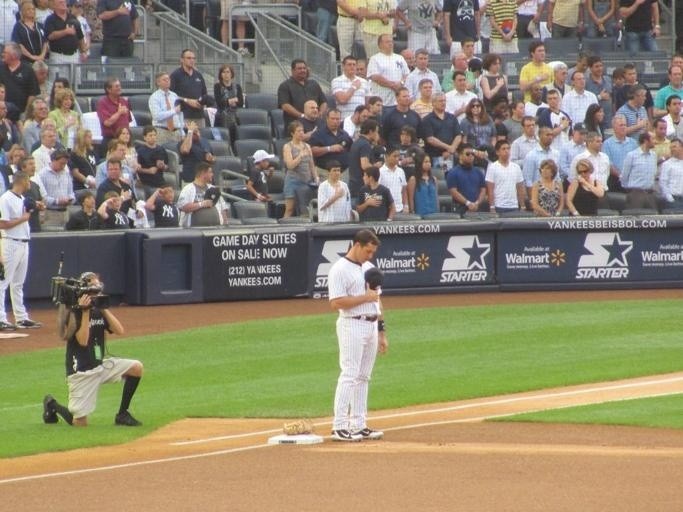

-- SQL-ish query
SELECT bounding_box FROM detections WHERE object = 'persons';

[44,272,144,427]
[0,1,275,232]
[278,0,683,233]
[327,229,387,442]
[0,172,43,331]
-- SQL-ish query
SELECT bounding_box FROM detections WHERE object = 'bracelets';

[378,321,386,331]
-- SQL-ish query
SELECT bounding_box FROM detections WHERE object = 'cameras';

[23,196,36,212]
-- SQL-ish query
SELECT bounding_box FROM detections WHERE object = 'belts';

[13,239,29,242]
[353,316,378,321]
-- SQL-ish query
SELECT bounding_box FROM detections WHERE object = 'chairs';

[304,11,670,85]
[73,93,683,220]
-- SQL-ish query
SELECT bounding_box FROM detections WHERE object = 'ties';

[166,96,174,130]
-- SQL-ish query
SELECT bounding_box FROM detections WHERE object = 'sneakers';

[116,411,139,425]
[43,395,58,422]
[0,319,40,330]
[332,428,383,441]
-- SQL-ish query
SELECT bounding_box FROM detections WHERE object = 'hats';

[253,150,274,163]
